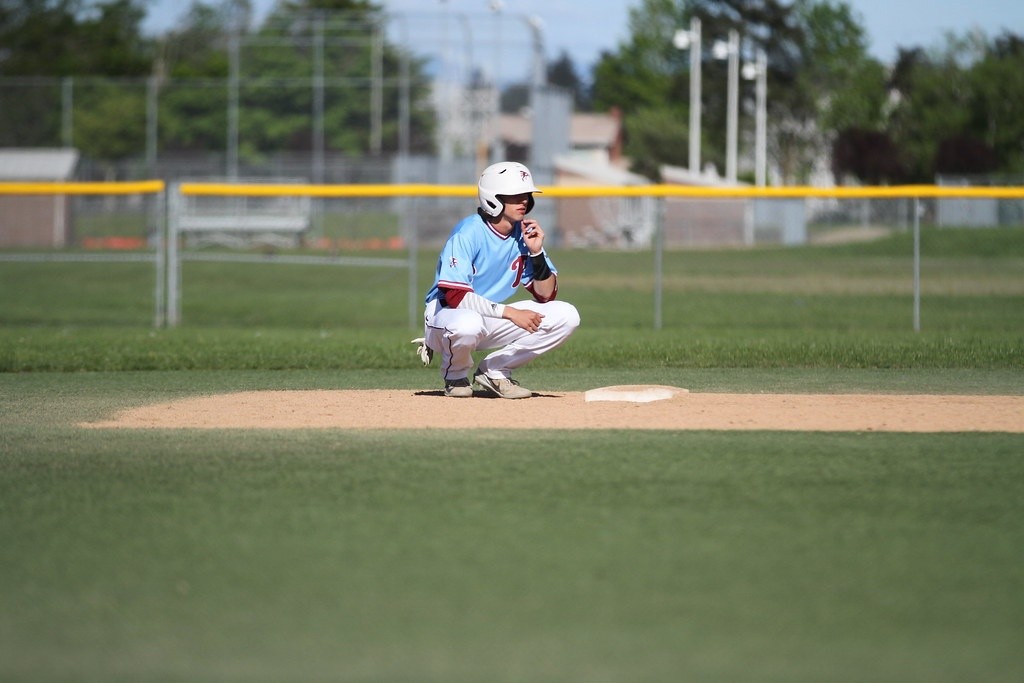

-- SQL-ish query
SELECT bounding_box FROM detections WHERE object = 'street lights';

[673,30,701,184]
[742,61,766,185]
[713,39,737,185]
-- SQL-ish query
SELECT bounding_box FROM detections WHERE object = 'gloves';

[410,338,433,366]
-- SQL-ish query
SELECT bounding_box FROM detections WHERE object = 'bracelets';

[530,251,552,281]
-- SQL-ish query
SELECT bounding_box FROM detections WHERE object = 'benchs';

[180,176,311,252]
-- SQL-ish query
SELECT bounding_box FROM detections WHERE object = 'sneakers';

[474,367,532,399]
[444,377,472,397]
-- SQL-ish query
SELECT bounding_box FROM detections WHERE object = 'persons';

[411,161,580,398]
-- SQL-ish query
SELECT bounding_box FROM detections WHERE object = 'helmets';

[478,162,543,217]
